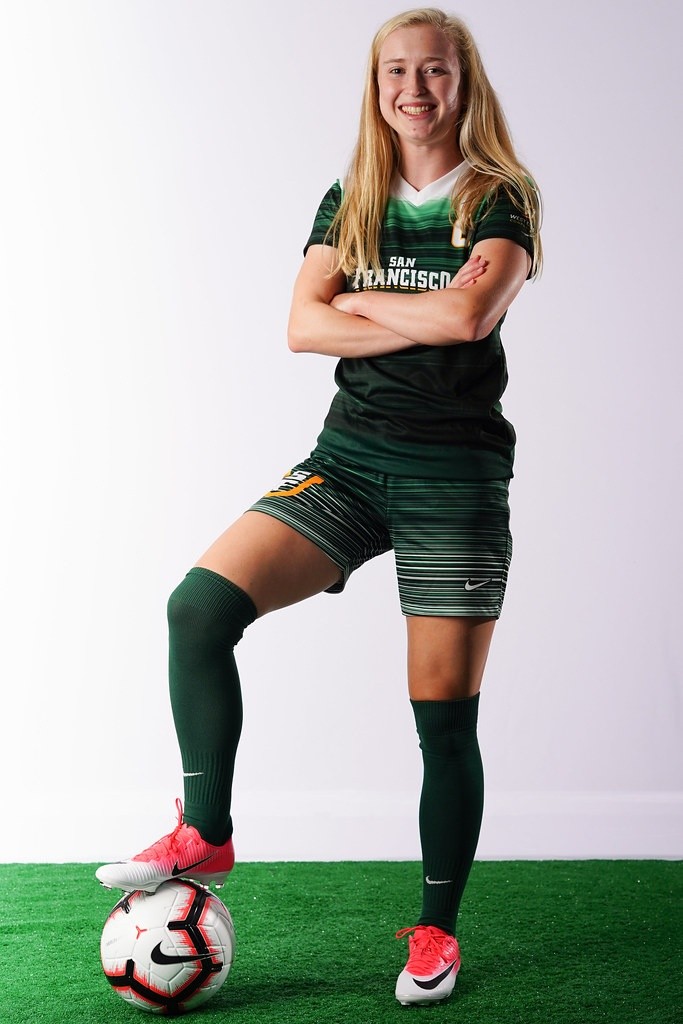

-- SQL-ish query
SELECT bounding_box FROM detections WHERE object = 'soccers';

[100,877,236,1014]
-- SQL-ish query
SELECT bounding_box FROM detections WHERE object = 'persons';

[93,7,539,1006]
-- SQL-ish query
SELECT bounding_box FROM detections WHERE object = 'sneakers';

[95,822,235,896]
[394,926,461,1007]
